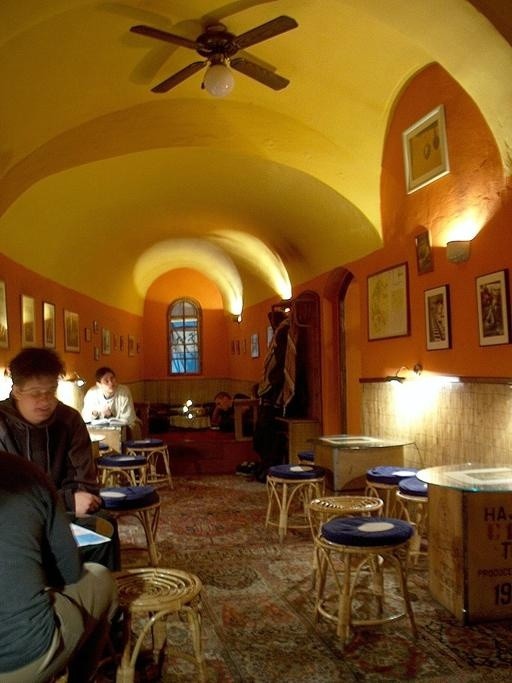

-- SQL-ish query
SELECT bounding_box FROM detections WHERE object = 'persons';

[78,367,138,428]
[1,449,120,683]
[0,346,122,571]
[209,381,262,437]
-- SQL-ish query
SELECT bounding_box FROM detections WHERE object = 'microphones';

[95,438,174,567]
[111,568,209,683]
[265,451,428,655]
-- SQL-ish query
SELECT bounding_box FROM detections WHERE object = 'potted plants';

[250,325,273,359]
[414,231,434,276]
[423,283,451,351]
[21,292,80,353]
[367,261,410,341]
[1,280,10,350]
[475,269,510,347]
[401,104,450,195]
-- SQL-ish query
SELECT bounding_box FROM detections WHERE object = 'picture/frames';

[306,434,415,498]
[66,511,120,572]
[415,462,512,626]
[86,422,126,474]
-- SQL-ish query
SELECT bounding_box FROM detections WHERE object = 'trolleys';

[129,15,299,93]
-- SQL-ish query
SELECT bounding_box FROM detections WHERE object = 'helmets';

[392,470,417,478]
[99,490,127,501]
[118,439,152,461]
[289,464,313,472]
[358,521,395,532]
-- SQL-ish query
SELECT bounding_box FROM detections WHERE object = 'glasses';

[203,58,235,97]
